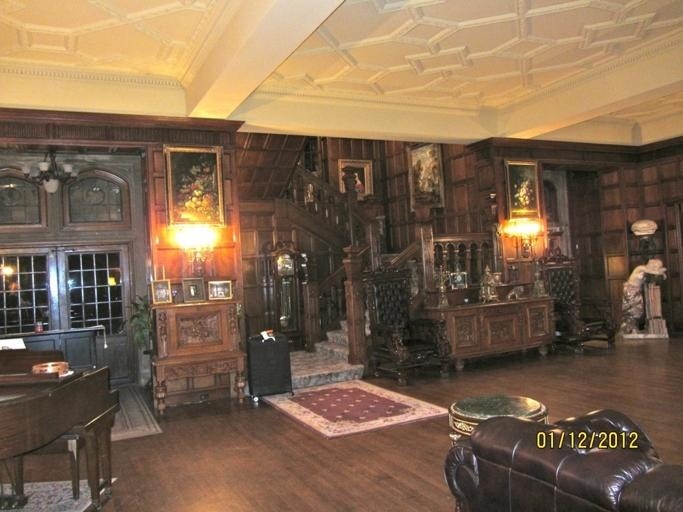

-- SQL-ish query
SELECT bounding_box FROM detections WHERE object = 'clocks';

[270,241,303,338]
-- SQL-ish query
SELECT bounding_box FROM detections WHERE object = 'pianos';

[0,349,120,511]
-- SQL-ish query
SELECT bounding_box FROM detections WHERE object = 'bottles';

[34,321,43,333]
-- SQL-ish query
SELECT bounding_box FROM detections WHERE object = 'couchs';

[444,408,682,509]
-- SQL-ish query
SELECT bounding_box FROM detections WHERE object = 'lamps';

[510,223,537,258]
[22,151,78,196]
[175,225,218,277]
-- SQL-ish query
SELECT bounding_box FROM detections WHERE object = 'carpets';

[262,379,449,441]
[0,475,118,512]
[110,383,162,443]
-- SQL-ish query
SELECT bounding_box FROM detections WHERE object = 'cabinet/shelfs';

[19,331,97,370]
[424,295,561,371]
[149,300,248,414]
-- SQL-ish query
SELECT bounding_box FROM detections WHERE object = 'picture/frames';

[181,278,206,304]
[162,143,224,230]
[337,156,374,202]
[503,156,544,218]
[150,278,173,305]
[405,142,445,213]
[208,280,233,301]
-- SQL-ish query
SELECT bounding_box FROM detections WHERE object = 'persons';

[618,259,668,334]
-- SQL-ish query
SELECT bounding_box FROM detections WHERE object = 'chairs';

[362,267,452,386]
[542,243,618,355]
